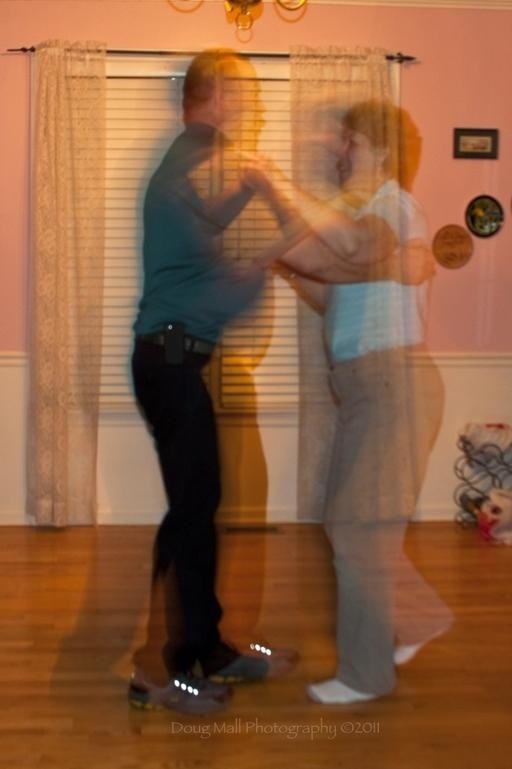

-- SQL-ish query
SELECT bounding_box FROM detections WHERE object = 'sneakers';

[128,642,270,715]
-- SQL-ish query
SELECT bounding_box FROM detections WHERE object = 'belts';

[144,331,214,355]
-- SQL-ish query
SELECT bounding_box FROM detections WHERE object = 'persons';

[126,47,455,715]
